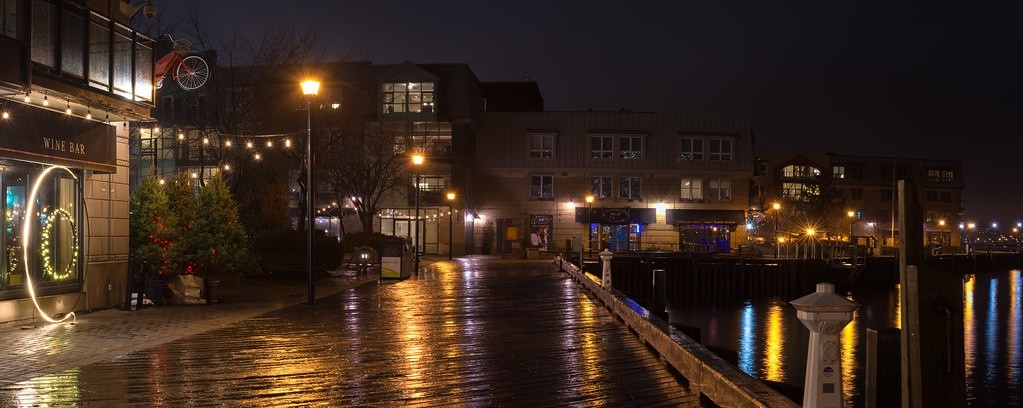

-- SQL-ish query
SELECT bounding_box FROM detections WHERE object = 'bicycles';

[154,33,212,93]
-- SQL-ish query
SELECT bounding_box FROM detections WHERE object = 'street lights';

[939,220,944,239]
[774,203,780,259]
[298,79,321,303]
[848,211,855,245]
[447,193,455,259]
[586,196,594,258]
[414,155,423,274]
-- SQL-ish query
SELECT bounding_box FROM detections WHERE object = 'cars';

[818,230,849,242]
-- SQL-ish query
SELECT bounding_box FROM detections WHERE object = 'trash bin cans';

[380,238,412,279]
